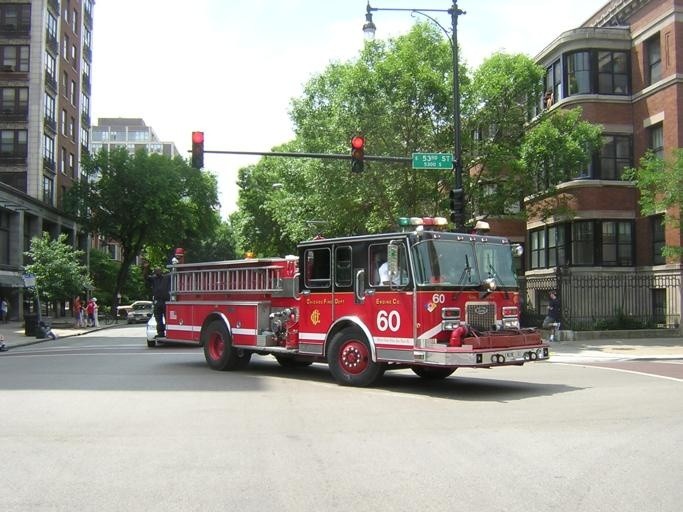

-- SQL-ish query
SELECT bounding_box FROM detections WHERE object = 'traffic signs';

[412,153,452,169]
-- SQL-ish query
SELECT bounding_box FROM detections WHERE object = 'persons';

[74,295,101,328]
[36,321,56,340]
[111,302,119,324]
[378,261,408,287]
[1,296,8,323]
[142,260,171,339]
[543,91,553,108]
[542,290,562,342]
[0,336,8,352]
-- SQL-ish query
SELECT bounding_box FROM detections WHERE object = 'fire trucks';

[155,215,551,386]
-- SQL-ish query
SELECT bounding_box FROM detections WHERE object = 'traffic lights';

[189,130,205,170]
[350,136,364,173]
[449,188,464,224]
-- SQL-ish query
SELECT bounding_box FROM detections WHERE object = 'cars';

[146,313,165,347]
[127,303,154,324]
[116,301,153,317]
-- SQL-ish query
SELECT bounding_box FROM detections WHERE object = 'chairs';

[371,251,390,285]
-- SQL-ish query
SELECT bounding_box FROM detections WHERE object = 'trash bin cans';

[23,314,37,336]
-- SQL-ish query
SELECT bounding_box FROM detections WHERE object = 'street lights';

[362,1,465,232]
